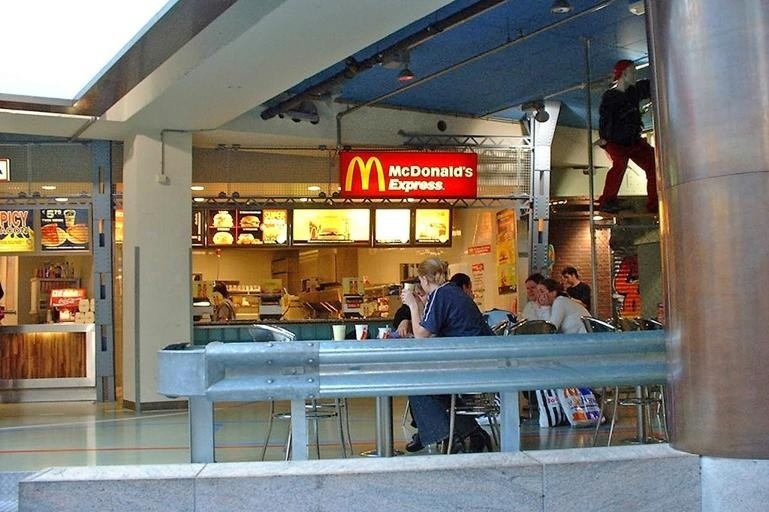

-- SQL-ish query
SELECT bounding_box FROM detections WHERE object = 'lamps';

[530,97,551,125]
[392,47,418,85]
[551,1,574,20]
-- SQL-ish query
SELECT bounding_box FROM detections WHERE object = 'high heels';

[444,434,465,454]
[469,430,494,452]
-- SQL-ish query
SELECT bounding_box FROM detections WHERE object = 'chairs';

[248,323,355,463]
[403,315,667,453]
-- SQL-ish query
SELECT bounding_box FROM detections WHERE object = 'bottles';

[34,260,76,278]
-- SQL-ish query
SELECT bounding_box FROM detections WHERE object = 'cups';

[378,328,391,339]
[63,209,76,228]
[404,283,415,293]
[354,324,368,340]
[331,325,347,341]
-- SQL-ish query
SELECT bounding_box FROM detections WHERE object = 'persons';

[210,283,236,321]
[450,272,477,303]
[518,272,551,410]
[559,266,593,311]
[534,275,593,335]
[386,277,429,430]
[399,256,495,454]
[594,57,661,214]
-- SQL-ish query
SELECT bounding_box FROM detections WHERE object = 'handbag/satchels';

[515,320,557,334]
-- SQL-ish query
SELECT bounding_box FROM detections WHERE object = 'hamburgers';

[212,213,233,228]
[213,232,234,244]
[239,216,260,228]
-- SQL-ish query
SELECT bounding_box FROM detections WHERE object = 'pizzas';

[66,224,90,243]
[41,223,60,244]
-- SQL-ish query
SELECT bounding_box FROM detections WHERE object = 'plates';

[41,226,67,247]
[65,224,89,245]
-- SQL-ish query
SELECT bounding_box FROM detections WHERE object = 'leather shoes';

[405,433,426,452]
